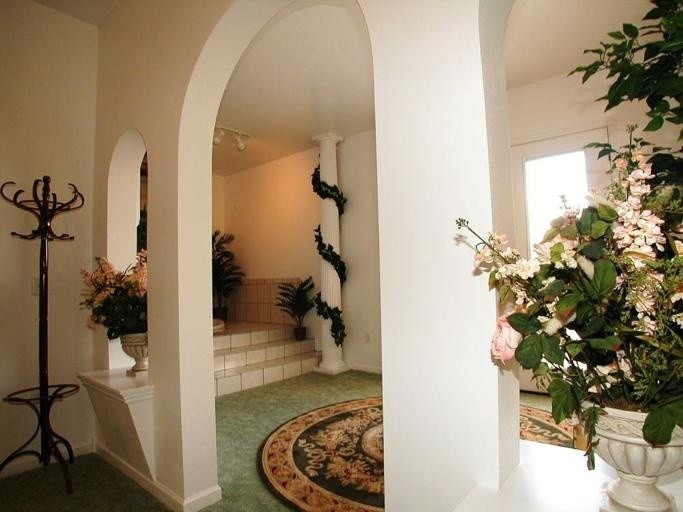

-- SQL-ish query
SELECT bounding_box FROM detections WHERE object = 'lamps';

[212,120,247,151]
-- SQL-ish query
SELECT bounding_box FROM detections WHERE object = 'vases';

[576,387,683,512]
[122,327,151,374]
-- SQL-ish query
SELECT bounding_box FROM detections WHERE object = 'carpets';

[253,394,585,511]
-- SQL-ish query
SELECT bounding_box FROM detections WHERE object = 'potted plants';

[212,229,245,324]
[273,276,319,341]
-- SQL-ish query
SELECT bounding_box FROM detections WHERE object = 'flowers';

[80,244,157,340]
[451,118,683,465]
